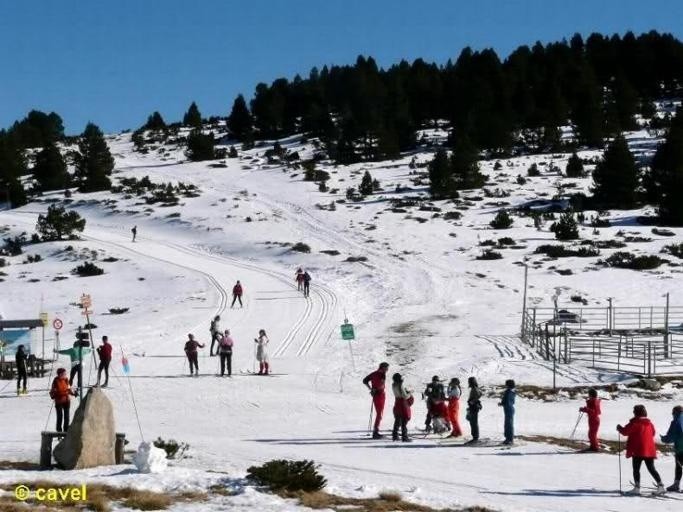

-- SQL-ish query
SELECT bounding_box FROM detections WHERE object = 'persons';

[254,329,269,375]
[660,406,683,491]
[49,369,76,442]
[422,375,452,432]
[616,405,665,496]
[498,380,515,444]
[391,373,413,442]
[466,377,482,443]
[209,315,223,356]
[295,268,303,275]
[442,378,463,438]
[184,334,205,377]
[131,226,137,242]
[362,363,389,439]
[303,271,311,296]
[230,281,243,308]
[579,389,601,451]
[295,273,304,291]
[217,329,233,377]
[52,343,95,394]
[16,345,29,396]
[93,337,112,388]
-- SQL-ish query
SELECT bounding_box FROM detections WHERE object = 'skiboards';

[240,369,288,376]
[592,490,683,502]
[629,482,683,493]
[1,392,31,397]
[84,384,115,389]
[357,427,453,440]
[556,450,616,454]
[437,439,527,450]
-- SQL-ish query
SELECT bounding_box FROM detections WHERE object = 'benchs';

[39,430,126,467]
